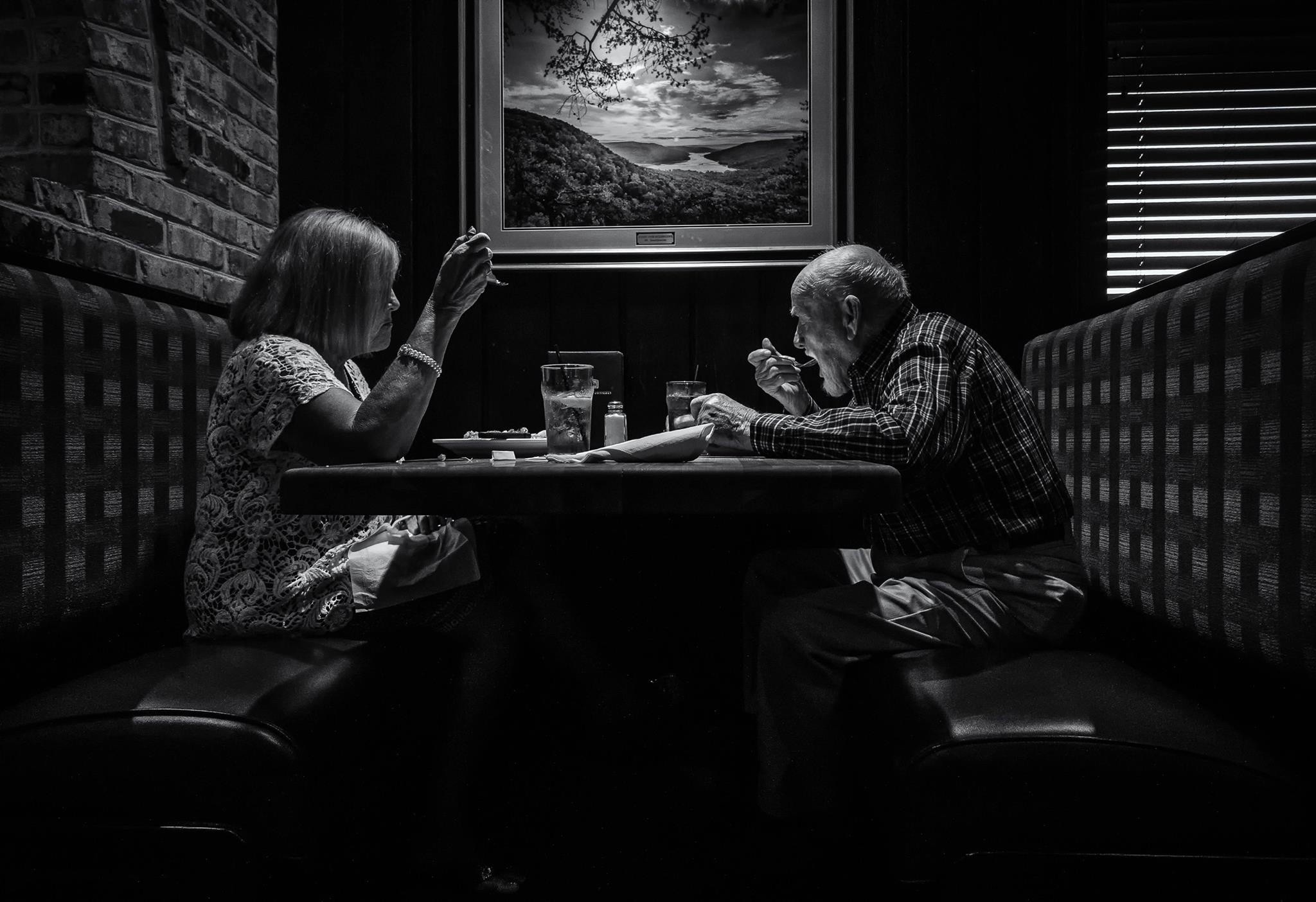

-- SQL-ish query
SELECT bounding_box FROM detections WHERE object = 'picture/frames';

[457,0,854,269]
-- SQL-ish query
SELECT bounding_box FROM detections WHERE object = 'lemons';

[674,414,695,428]
[558,397,593,408]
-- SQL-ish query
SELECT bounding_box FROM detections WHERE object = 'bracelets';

[398,343,442,377]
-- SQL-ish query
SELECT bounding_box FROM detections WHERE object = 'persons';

[688,243,1091,834]
[182,203,500,819]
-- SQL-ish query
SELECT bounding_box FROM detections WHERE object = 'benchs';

[0,266,411,902]
[843,216,1316,902]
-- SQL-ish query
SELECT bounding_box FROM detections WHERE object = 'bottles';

[604,400,628,447]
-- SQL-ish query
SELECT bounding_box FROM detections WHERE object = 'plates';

[432,438,547,456]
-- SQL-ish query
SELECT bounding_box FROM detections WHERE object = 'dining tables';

[280,452,897,902]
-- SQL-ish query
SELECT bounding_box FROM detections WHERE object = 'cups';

[666,380,707,432]
[540,362,595,455]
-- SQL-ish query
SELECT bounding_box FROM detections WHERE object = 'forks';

[464,226,509,287]
[770,355,817,368]
